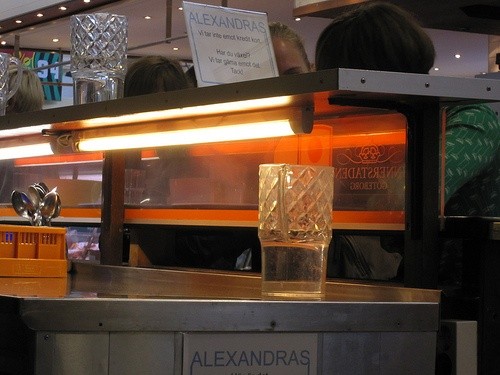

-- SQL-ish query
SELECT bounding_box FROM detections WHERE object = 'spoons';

[9,181,61,226]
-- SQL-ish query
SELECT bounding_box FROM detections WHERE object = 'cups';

[256,163,334,301]
[68,14,128,107]
[0,53,23,116]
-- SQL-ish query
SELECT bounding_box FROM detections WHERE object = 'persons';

[6,64,44,115]
[265,21,405,281]
[122,55,263,273]
[315,2,500,321]
